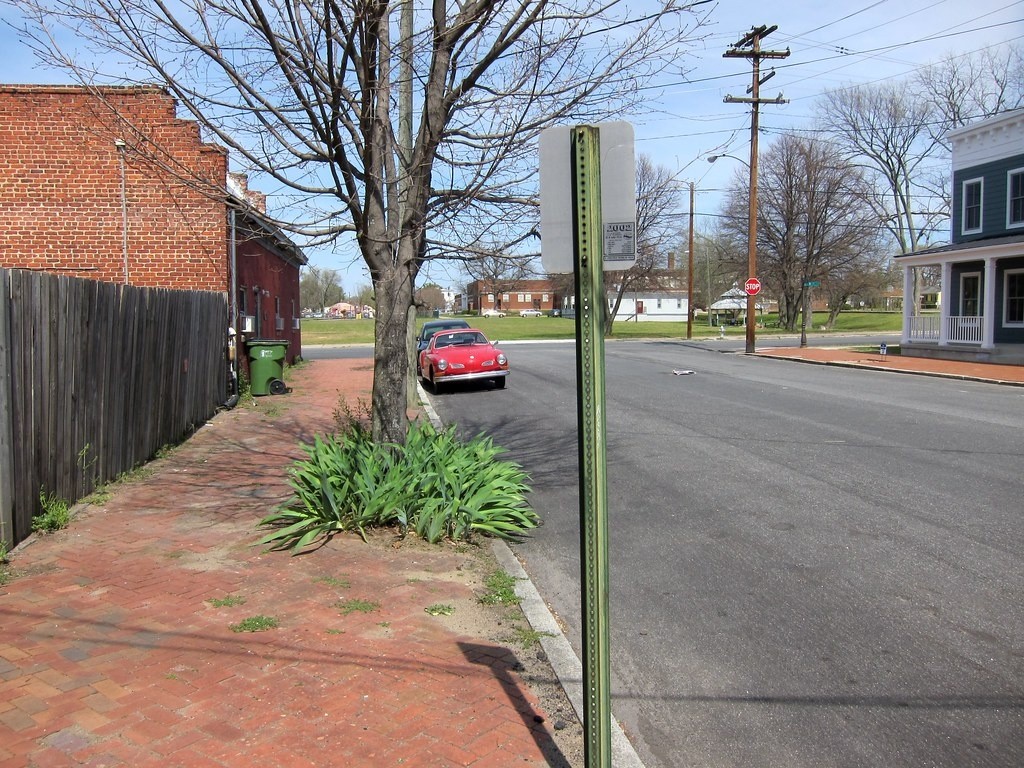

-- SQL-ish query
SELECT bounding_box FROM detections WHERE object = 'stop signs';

[745,278,761,295]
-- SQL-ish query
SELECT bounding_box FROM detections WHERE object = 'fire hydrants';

[719,326,725,338]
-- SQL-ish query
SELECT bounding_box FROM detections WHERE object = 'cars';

[519,309,543,318]
[315,313,322,317]
[415,319,470,375]
[547,309,561,317]
[419,329,510,395]
[482,310,506,319]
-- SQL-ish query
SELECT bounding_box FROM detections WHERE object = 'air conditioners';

[275,318,285,331]
[239,315,256,333]
[292,318,300,330]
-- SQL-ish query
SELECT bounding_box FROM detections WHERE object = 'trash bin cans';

[245,339,289,397]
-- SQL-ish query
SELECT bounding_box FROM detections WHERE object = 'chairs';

[464,339,474,344]
[435,343,449,348]
[425,331,436,342]
